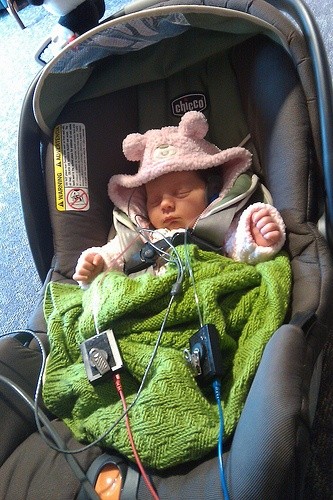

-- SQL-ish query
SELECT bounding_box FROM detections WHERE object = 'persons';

[72,111,283,433]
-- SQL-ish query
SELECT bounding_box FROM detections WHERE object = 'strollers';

[0,0,332,500]
